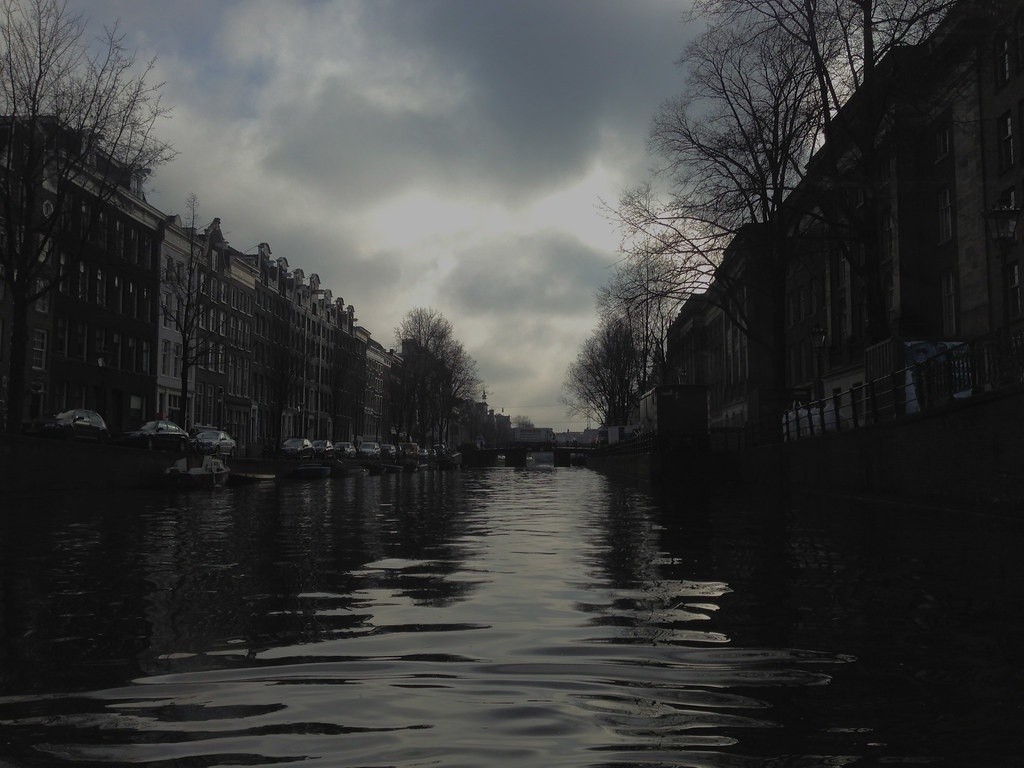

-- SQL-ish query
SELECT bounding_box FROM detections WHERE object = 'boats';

[345,462,430,478]
[288,463,332,481]
[159,456,231,490]
[526,456,536,463]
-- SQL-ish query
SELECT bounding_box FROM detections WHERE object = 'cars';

[380,444,397,458]
[21,409,113,446]
[334,442,356,459]
[398,442,449,459]
[359,442,382,460]
[189,431,238,458]
[278,438,317,461]
[311,440,336,459]
[121,419,191,450]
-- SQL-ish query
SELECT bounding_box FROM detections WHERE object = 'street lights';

[977,197,1023,383]
[808,323,828,400]
